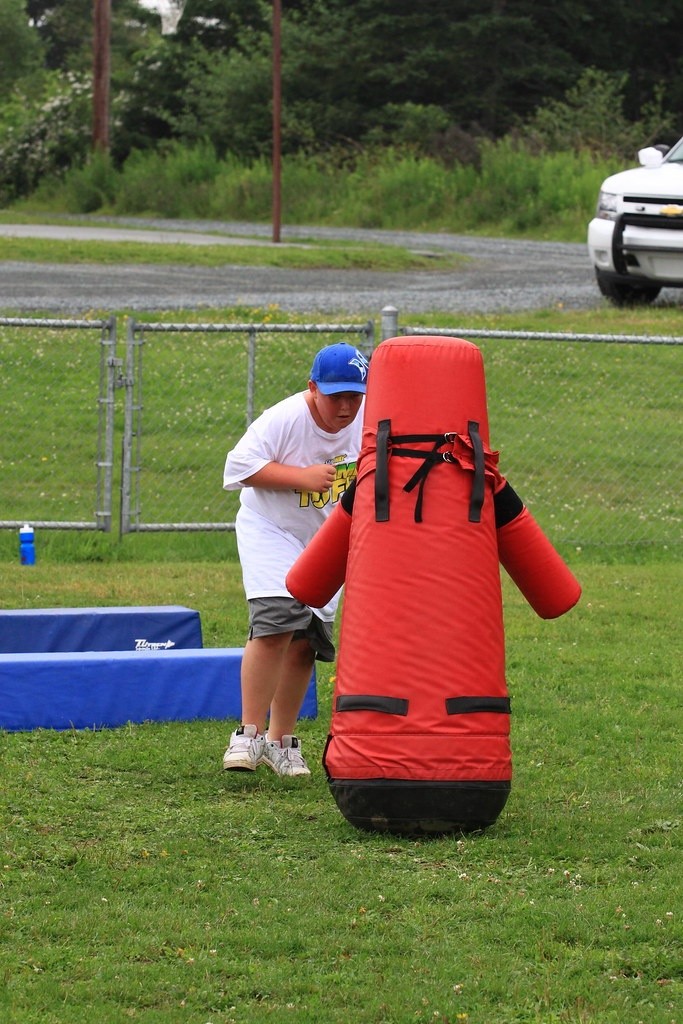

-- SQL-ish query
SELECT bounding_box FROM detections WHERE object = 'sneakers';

[262,731,310,776]
[224,723,262,773]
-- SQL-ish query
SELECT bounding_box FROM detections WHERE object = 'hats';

[311,343,367,395]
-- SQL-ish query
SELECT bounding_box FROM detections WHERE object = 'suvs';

[585,135,683,308]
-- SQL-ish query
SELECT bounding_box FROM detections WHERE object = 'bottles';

[20,522,35,566]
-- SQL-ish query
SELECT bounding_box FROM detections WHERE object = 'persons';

[220,340,370,778]
